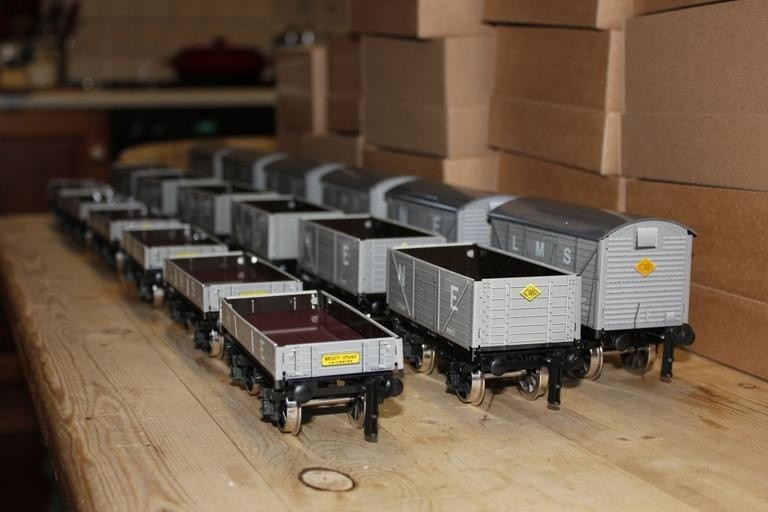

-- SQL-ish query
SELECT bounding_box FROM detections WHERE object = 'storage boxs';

[479,1,766,386]
[277,2,498,191]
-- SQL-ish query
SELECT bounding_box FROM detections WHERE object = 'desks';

[2,212,768,511]
